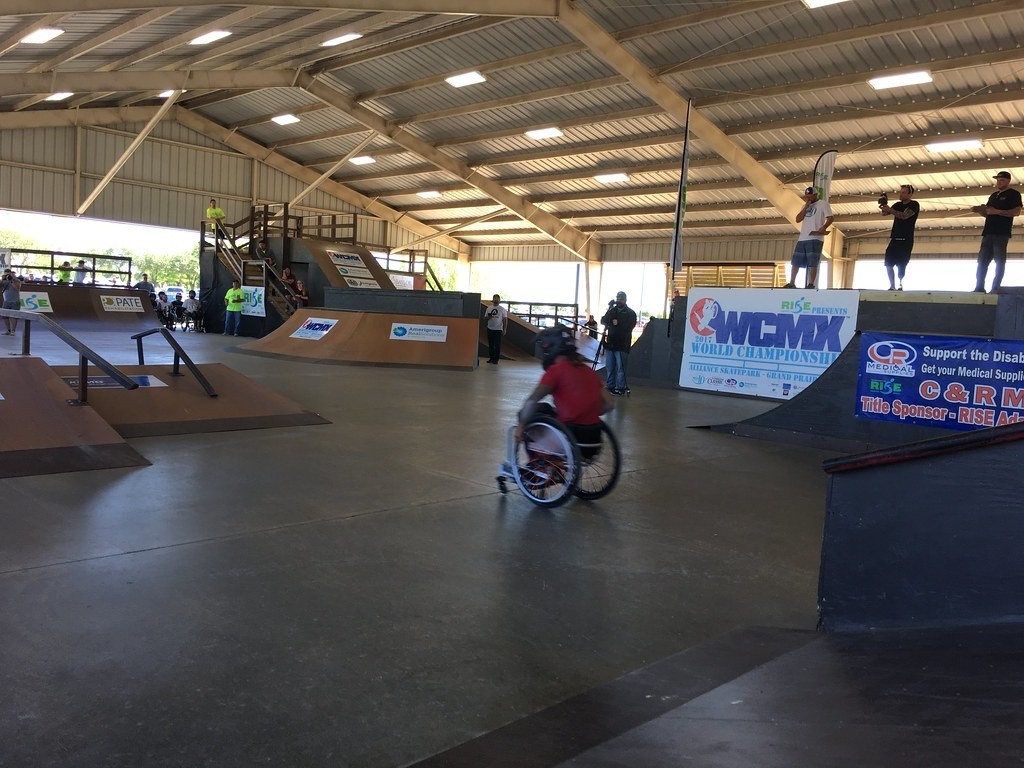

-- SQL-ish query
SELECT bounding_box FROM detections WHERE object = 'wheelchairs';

[180,310,205,332]
[495,403,622,509]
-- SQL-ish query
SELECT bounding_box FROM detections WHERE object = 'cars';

[162,286,186,303]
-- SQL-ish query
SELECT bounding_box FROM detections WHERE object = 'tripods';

[591,306,631,393]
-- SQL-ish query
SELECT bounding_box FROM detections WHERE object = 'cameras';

[877,193,888,207]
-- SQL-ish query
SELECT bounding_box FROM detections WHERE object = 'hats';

[176,293,182,297]
[992,171,1011,179]
[616,291,626,297]
[78,261,84,263]
[805,187,817,197]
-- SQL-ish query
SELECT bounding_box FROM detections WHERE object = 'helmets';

[535,324,578,369]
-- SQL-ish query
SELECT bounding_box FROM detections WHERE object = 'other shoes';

[487,358,498,364]
[783,283,796,288]
[222,333,229,336]
[604,385,625,396]
[234,333,238,337]
[285,308,289,312]
[989,290,999,294]
[2,330,11,335]
[10,330,15,336]
[971,290,986,294]
[272,290,275,296]
[807,283,815,288]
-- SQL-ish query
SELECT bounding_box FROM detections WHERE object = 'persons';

[222,280,246,337]
[57,261,73,286]
[600,292,637,396]
[0,269,21,336]
[498,324,615,480]
[483,294,507,364]
[206,199,226,248]
[127,273,156,293]
[72,260,91,286]
[783,187,834,289]
[670,290,680,321]
[11,271,48,284]
[256,240,277,296]
[181,290,203,331]
[581,315,597,340]
[279,267,309,316]
[149,290,183,331]
[879,185,920,291]
[970,171,1023,293]
